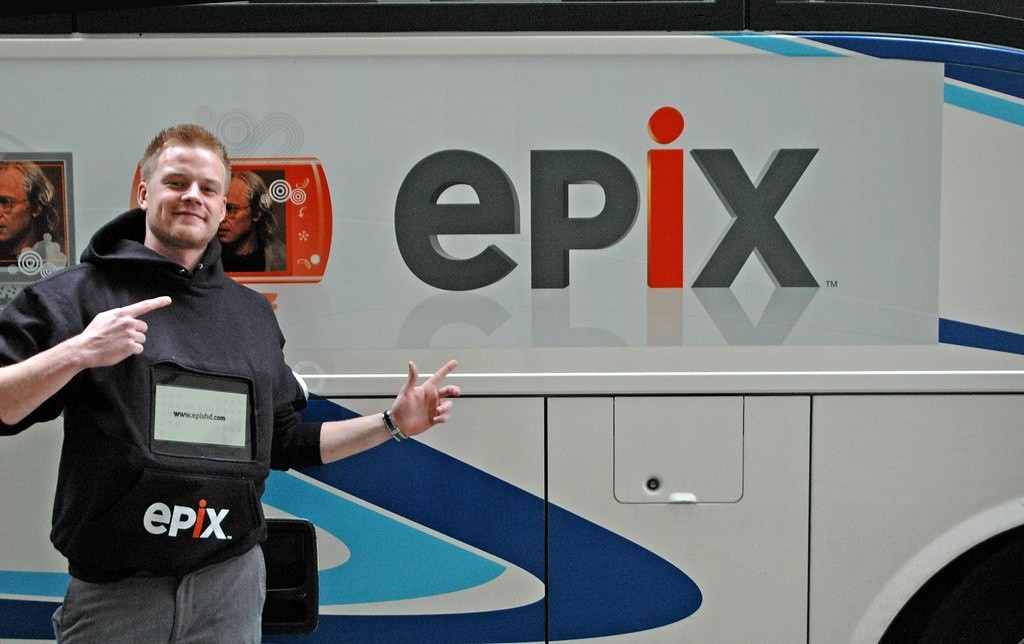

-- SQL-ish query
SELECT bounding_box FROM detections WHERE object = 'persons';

[0,162,59,261]
[0,124,461,644]
[217,171,288,272]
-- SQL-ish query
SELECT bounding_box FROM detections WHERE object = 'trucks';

[0,28,1024,644]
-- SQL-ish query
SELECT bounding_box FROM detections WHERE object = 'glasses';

[0,197,33,212]
[225,202,254,215]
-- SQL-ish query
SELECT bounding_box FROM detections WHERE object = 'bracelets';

[381,411,408,442]
